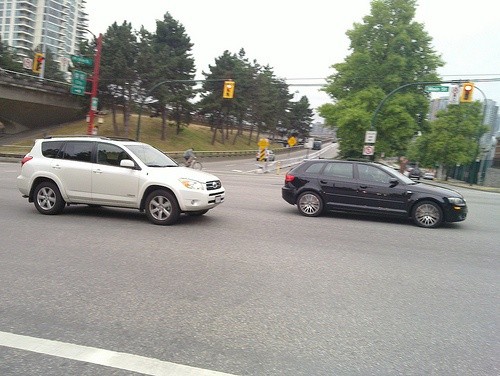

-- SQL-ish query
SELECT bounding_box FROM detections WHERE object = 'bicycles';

[177,156,202,172]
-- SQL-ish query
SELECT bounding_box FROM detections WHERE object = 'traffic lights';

[33,52,45,75]
[460,82,474,103]
[222,80,235,99]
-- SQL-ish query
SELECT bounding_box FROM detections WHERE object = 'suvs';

[17,135,226,225]
[281,158,468,229]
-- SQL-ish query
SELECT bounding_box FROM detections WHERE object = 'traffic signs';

[72,71,87,96]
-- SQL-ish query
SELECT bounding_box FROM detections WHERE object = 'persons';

[399,157,410,174]
[183,148,194,163]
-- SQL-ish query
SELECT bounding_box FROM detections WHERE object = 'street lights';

[77,25,102,134]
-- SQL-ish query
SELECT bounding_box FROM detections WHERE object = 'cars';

[257,149,276,161]
[333,138,340,143]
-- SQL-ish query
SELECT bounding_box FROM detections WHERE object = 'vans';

[311,141,322,150]
